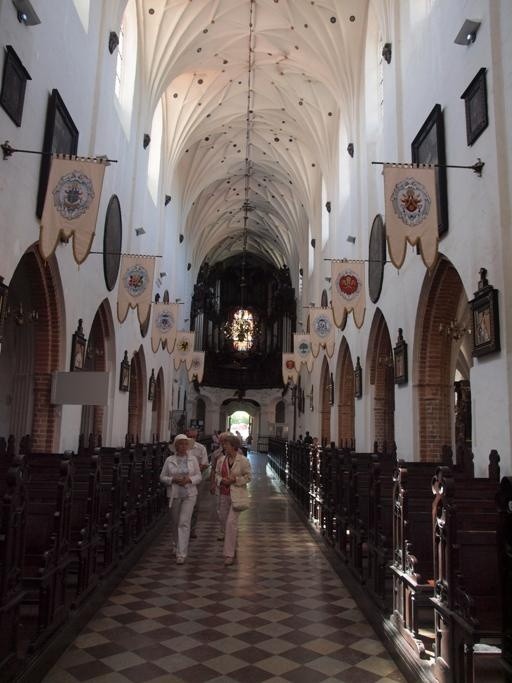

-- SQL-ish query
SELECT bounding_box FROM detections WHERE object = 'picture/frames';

[353,368,363,397]
[328,384,335,404]
[148,378,156,400]
[462,68,489,145]
[393,343,407,384]
[119,362,132,392]
[410,104,450,255]
[69,334,87,371]
[467,289,501,357]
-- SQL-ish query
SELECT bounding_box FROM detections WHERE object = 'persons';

[303,430,313,444]
[207,432,234,541]
[214,433,252,564]
[299,434,304,444]
[185,424,253,455]
[160,433,203,564]
[177,428,209,538]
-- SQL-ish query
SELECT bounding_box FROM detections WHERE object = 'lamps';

[220,157,263,352]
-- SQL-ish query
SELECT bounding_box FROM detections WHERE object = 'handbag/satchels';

[230,483,249,511]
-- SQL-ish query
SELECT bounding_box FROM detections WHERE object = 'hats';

[168,434,193,452]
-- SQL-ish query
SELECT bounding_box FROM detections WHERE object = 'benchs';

[1,433,174,683]
[266,436,512,683]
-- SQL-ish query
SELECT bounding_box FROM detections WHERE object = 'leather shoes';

[217,530,224,539]
[176,557,185,564]
[224,557,233,565]
[191,530,197,539]
